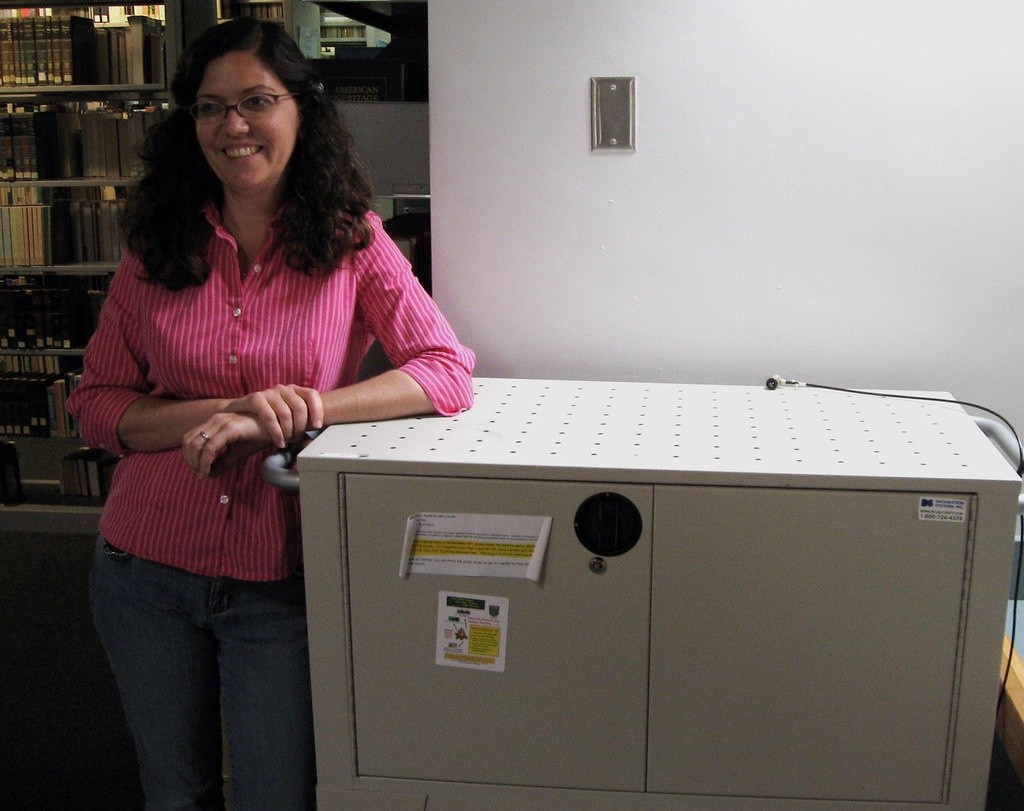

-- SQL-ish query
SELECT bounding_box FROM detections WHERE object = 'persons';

[66,16,476,811]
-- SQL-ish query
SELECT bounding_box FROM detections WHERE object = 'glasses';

[187,92,300,123]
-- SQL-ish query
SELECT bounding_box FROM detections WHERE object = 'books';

[320,7,366,58]
[0,5,166,498]
[241,3,284,29]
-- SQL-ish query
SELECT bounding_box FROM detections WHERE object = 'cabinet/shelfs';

[1,0,392,537]
[256,380,1024,811]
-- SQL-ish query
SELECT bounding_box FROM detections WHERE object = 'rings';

[199,429,210,441]
